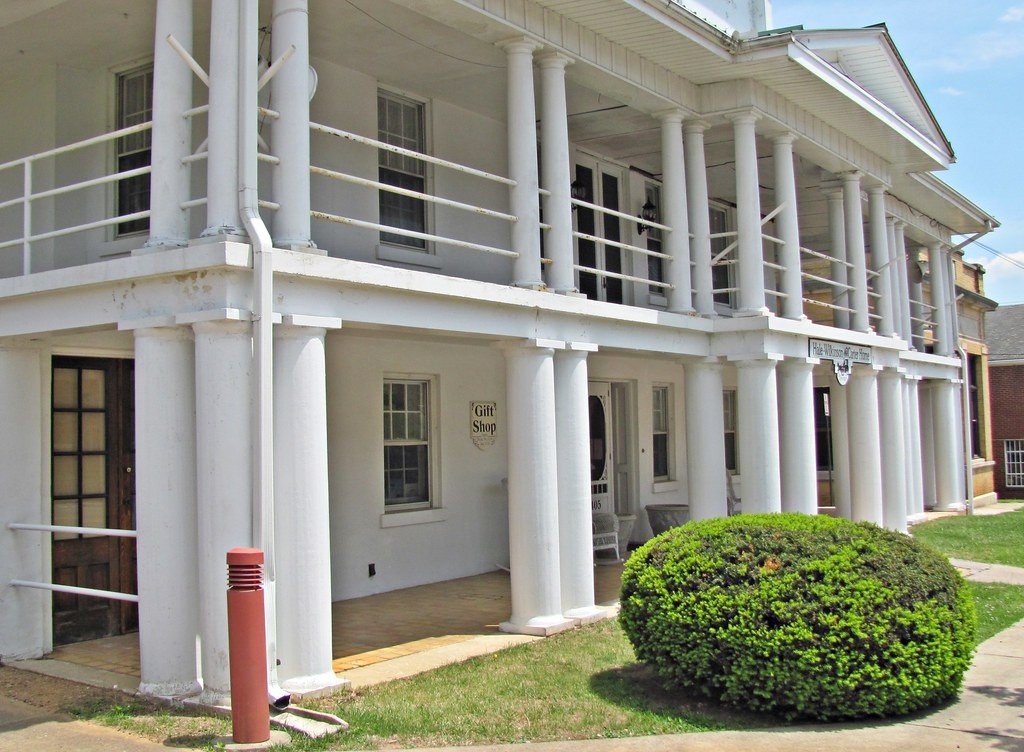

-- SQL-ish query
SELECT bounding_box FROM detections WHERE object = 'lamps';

[637,195,658,234]
[571,176,587,210]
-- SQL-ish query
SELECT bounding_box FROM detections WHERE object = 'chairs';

[725,467,741,517]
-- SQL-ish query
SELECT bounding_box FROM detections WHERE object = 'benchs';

[495,477,624,573]
